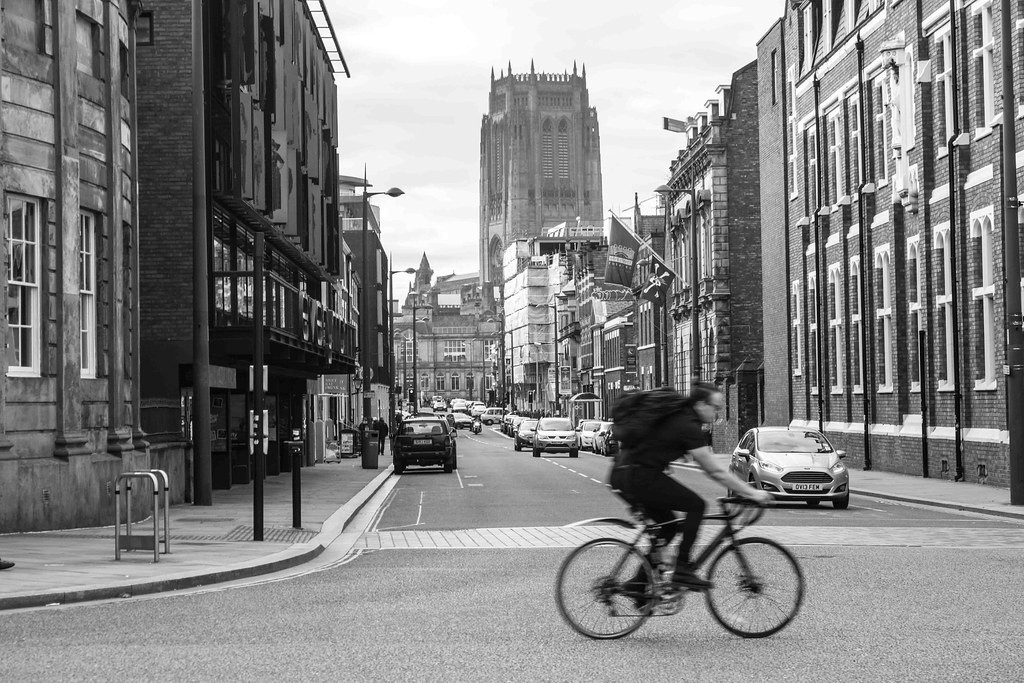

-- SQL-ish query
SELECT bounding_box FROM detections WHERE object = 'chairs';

[406,427,414,432]
[431,426,441,433]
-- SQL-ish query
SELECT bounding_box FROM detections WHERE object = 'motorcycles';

[472,420,481,436]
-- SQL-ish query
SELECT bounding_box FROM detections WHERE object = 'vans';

[480,407,510,426]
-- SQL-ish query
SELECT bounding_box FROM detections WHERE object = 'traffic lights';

[504,391,509,405]
[487,390,494,401]
[398,401,402,406]
[528,391,533,404]
[409,388,415,403]
[505,357,511,366]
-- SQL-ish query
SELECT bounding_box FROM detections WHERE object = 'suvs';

[531,416,582,457]
[393,412,457,473]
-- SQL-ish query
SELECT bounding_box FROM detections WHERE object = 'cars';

[576,419,617,457]
[430,395,488,418]
[446,413,472,428]
[727,426,850,509]
[499,411,539,451]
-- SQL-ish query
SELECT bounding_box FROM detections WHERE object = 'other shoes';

[670,570,713,588]
[622,577,654,616]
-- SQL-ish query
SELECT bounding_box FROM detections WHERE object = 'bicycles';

[553,496,804,640]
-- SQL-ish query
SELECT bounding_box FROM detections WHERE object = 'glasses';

[706,401,723,414]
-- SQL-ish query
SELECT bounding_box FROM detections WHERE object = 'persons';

[605,382,771,617]
[374,416,388,455]
[529,410,551,419]
[470,415,481,432]
[396,412,402,426]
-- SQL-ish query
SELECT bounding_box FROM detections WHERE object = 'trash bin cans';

[361,430,379,469]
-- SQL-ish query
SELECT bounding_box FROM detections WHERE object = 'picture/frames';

[136,10,155,46]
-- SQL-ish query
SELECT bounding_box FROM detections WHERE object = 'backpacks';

[611,385,692,443]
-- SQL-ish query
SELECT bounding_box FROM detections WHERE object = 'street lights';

[362,163,406,428]
[536,303,559,415]
[389,251,416,436]
[408,289,433,416]
[472,338,485,405]
[486,318,506,419]
[462,342,472,378]
